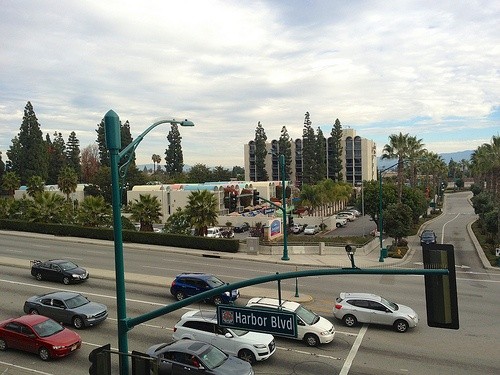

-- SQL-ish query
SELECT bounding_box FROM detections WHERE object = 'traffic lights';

[253,189,259,207]
[224,192,232,208]
[230,194,238,213]
[89,344,111,375]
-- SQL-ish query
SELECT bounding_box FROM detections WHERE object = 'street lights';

[378,159,427,262]
[104,109,194,375]
[268,152,290,260]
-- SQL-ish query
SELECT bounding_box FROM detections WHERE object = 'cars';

[0,315,82,361]
[145,339,255,375]
[170,272,240,306]
[30,259,90,285]
[134,222,235,239]
[233,222,249,233]
[23,292,109,330]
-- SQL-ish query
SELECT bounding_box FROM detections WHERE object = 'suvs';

[294,223,304,234]
[336,210,361,228]
[245,297,335,347]
[173,310,277,365]
[304,224,319,235]
[419,229,437,246]
[333,292,418,333]
